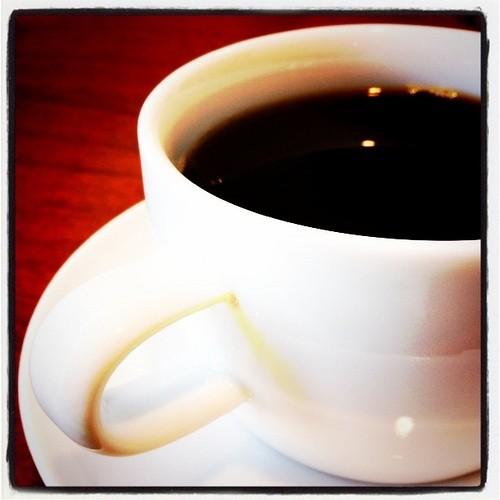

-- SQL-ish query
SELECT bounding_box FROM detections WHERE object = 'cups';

[135,22,483,482]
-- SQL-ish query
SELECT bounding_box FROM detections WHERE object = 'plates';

[18,197,482,486]
